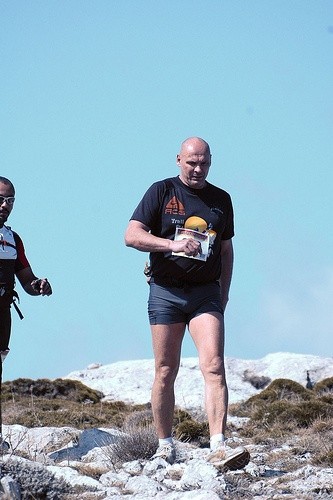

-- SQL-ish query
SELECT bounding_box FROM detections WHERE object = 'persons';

[0,177,52,454]
[124,136,251,474]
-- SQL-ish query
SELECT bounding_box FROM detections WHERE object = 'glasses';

[0,196,14,204]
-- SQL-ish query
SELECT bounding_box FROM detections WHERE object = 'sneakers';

[203,446,250,471]
[148,442,175,466]
